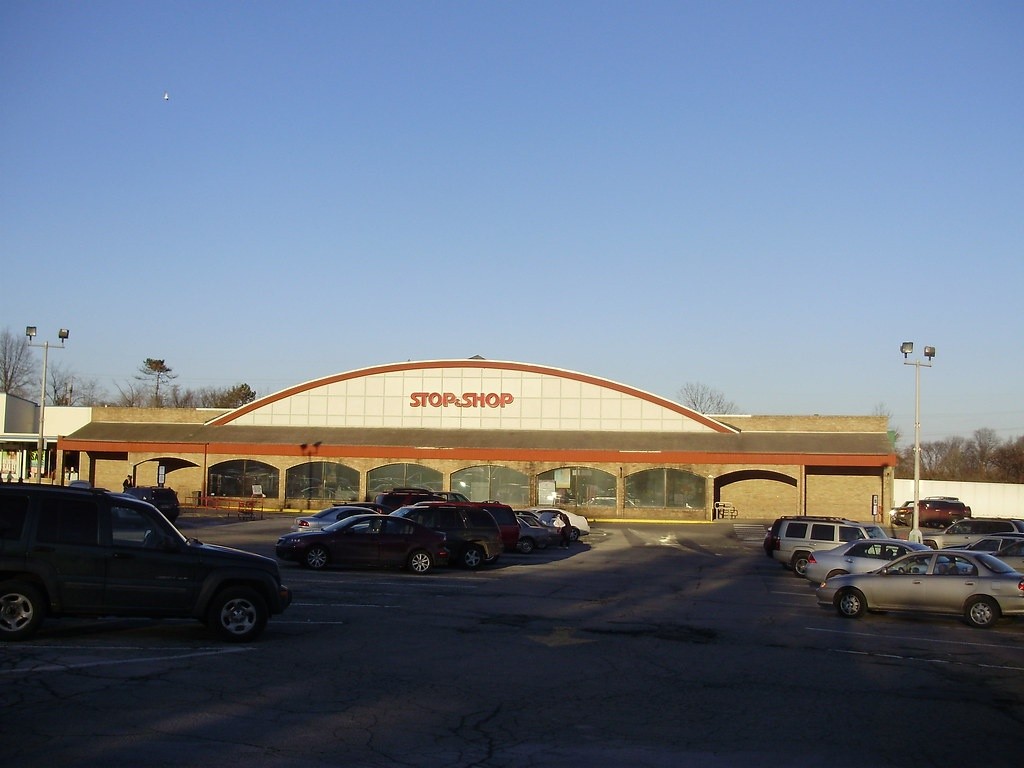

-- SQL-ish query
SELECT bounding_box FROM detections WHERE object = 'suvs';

[123,487,180,525]
[0,482,292,644]
[895,497,971,528]
[388,507,504,568]
[412,499,520,563]
[763,516,859,571]
[920,518,1024,550]
[371,486,471,513]
[773,519,910,578]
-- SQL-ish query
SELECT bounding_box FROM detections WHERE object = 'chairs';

[885,549,895,560]
[895,547,907,558]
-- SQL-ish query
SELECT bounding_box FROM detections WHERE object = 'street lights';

[26,326,69,484]
[900,341,935,545]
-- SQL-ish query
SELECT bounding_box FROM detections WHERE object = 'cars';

[274,514,447,575]
[295,473,638,508]
[332,502,395,515]
[513,510,561,554]
[989,540,1024,580]
[529,509,590,542]
[69,480,92,489]
[291,506,383,532]
[942,536,1024,550]
[816,550,1024,628]
[804,538,973,585]
